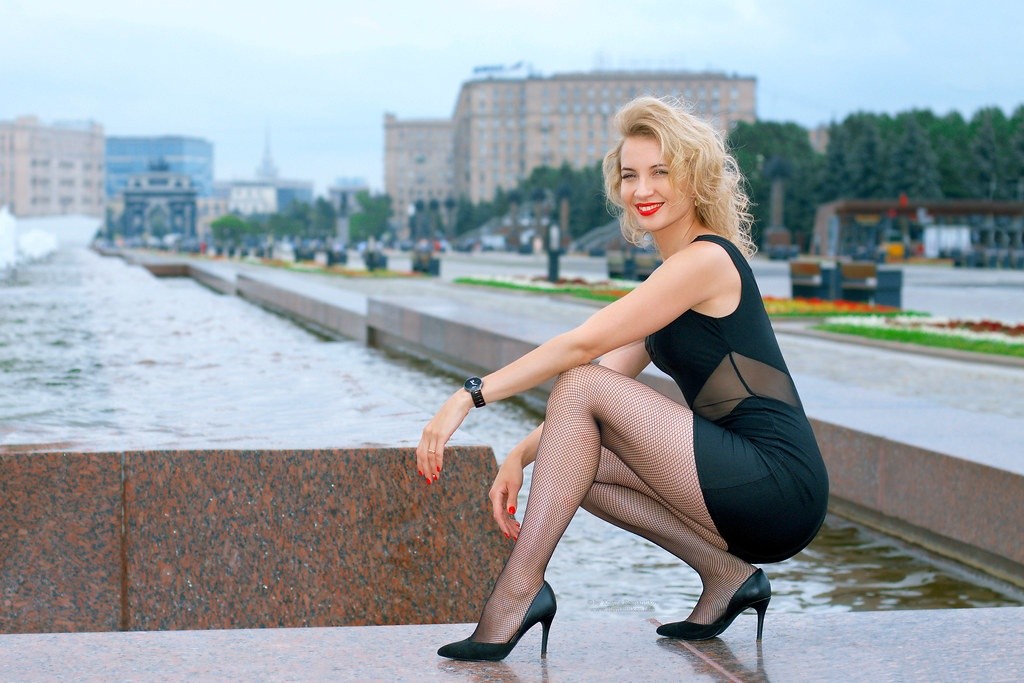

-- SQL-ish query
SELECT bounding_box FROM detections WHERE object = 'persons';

[414,97,829,662]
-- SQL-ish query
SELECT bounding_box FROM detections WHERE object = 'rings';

[428,450,435,454]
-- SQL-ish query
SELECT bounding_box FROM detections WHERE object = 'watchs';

[464,377,486,408]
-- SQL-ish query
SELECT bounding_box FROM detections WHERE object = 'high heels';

[437,580,556,661]
[656,568,772,641]
